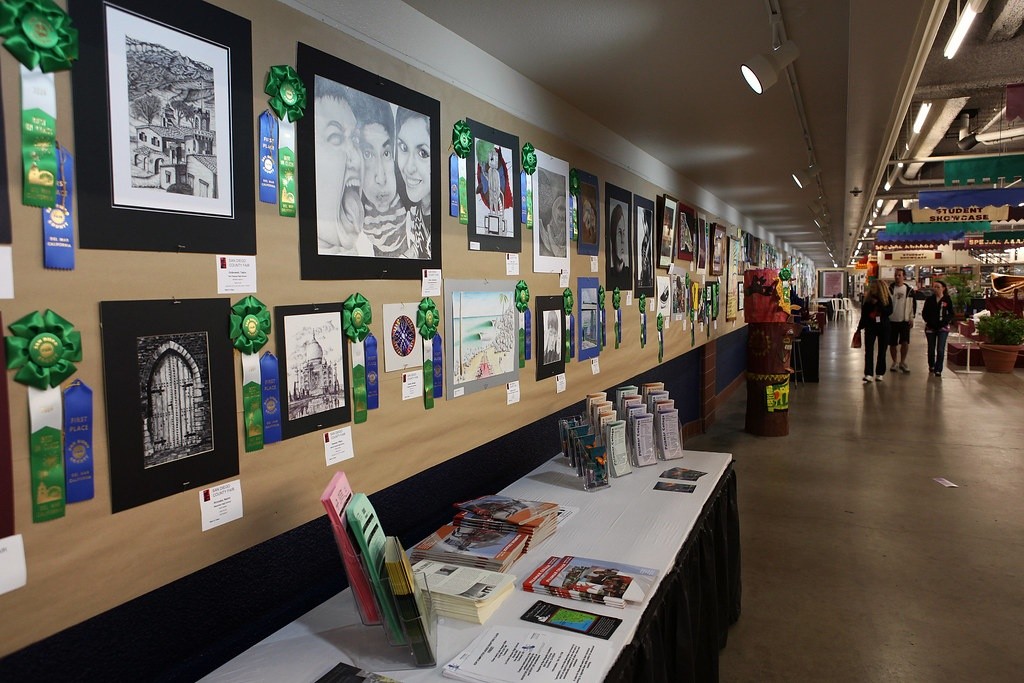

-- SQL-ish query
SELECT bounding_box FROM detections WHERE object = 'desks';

[811,298,831,323]
[197,450,743,683]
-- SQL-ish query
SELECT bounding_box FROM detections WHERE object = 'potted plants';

[975,310,1024,374]
[943,275,983,328]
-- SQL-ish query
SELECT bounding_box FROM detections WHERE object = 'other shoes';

[929,366,941,377]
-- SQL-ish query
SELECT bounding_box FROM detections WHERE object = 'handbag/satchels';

[851,330,861,348]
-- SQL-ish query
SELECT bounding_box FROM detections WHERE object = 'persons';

[888,269,917,371]
[857,280,893,382]
[922,281,954,376]
[826,293,844,321]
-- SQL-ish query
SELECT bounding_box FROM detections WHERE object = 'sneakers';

[890,360,898,371]
[863,375,873,382]
[875,375,883,381]
[899,362,910,372]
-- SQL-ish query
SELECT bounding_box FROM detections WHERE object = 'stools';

[792,339,805,389]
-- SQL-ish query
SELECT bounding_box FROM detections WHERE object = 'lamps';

[943,0,988,60]
[792,154,823,190]
[806,196,840,269]
[738,12,802,98]
[861,100,932,240]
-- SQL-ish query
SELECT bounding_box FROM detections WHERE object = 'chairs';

[831,298,858,323]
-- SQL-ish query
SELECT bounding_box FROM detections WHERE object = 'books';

[523,556,659,609]
[412,560,516,624]
[409,496,559,574]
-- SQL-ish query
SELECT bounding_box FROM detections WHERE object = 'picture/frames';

[604,180,761,322]
[68,0,259,256]
[532,147,572,275]
[573,168,601,255]
[535,294,565,381]
[273,302,353,441]
[465,120,523,252]
[577,277,600,361]
[99,297,240,514]
[296,42,440,281]
[443,279,519,401]
